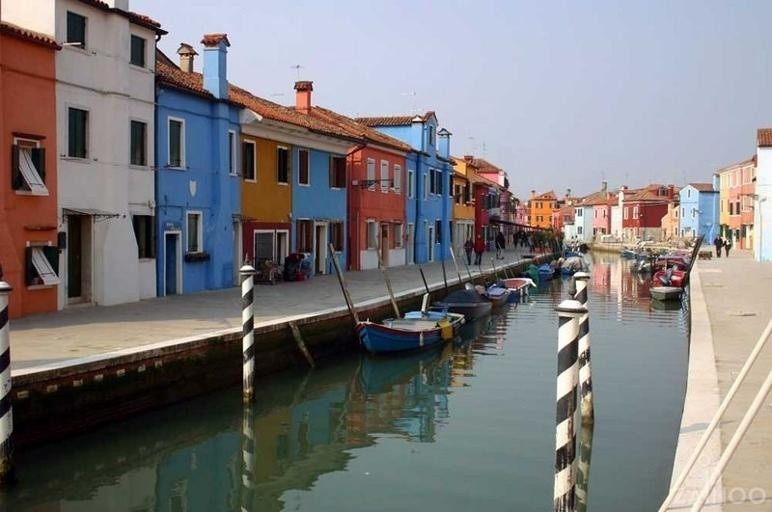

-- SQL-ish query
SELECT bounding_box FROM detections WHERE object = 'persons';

[713,234,723,258]
[473,234,486,265]
[463,237,475,265]
[723,235,732,258]
[513,230,543,252]
[495,232,505,260]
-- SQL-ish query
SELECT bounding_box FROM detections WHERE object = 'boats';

[519,247,590,281]
[620,247,690,301]
[355,278,533,352]
